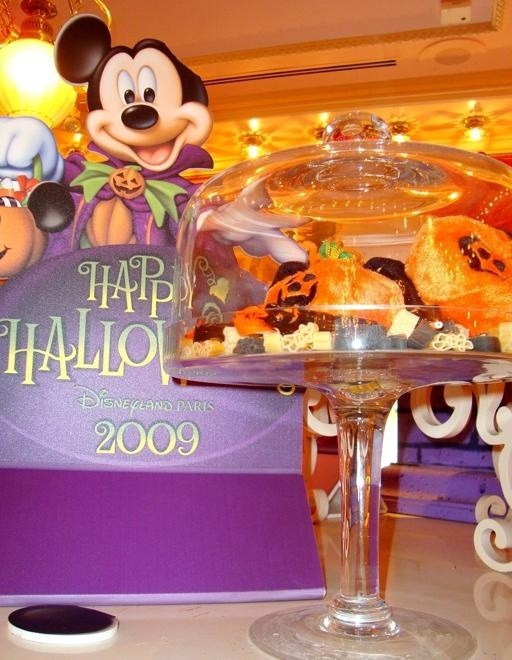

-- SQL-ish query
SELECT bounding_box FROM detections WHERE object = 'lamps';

[0,0,111,129]
